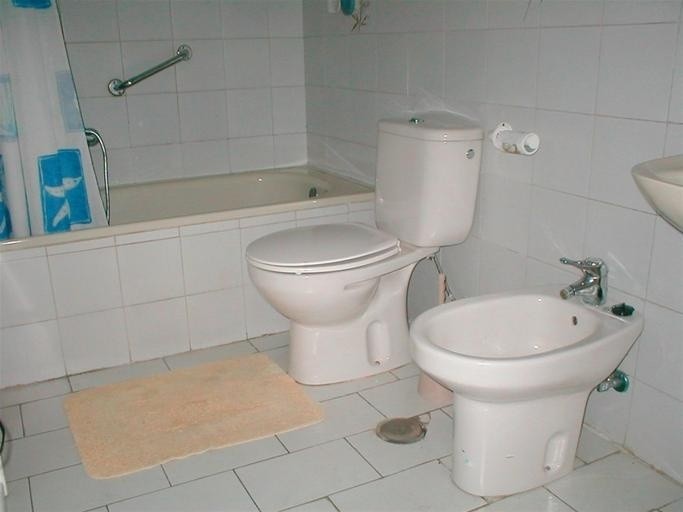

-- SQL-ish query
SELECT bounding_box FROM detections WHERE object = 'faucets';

[557,254,609,307]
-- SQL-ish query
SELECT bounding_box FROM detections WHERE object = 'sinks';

[631,155,683,234]
[409,292,646,496]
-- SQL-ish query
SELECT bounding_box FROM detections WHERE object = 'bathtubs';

[0,166,374,392]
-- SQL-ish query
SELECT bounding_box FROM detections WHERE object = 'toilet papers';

[497,130,538,159]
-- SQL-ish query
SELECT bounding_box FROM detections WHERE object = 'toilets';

[245,112,484,385]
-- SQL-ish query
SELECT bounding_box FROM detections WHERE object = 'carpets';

[61,349,328,483]
